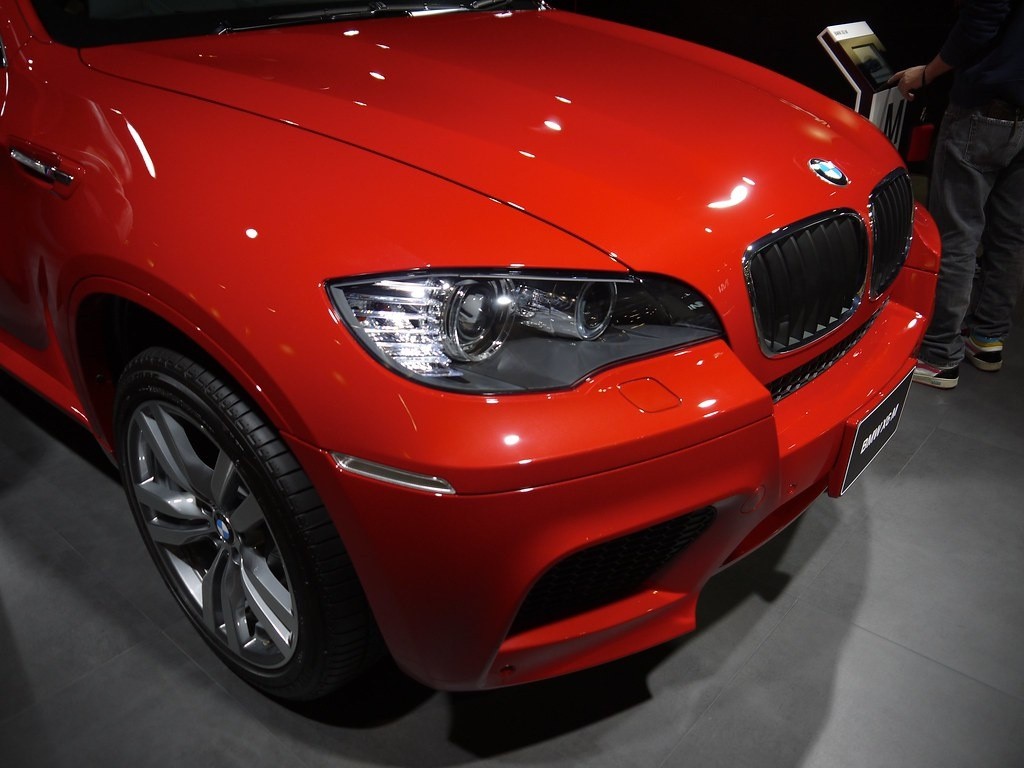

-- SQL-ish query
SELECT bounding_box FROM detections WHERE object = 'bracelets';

[922,66,927,86]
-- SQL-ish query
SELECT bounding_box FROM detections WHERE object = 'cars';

[0,0,942,723]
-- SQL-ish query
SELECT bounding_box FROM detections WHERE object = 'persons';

[887,0,1024,388]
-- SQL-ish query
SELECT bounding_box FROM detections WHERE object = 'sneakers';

[912,357,959,389]
[963,329,1002,370]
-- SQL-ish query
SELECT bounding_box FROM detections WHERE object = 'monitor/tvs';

[853,45,893,84]
